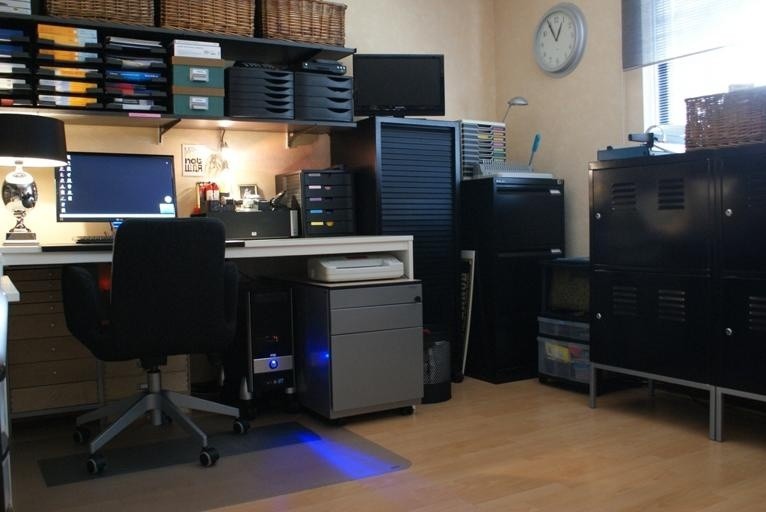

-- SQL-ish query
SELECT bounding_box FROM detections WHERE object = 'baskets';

[43,0,155,27]
[258,0,347,46]
[160,0,256,38]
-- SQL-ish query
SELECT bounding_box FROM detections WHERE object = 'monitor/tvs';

[352,53,447,120]
[55,151,179,232]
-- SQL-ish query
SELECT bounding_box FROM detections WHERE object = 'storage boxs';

[538,313,590,341]
[168,86,224,117]
[537,336,590,385]
[167,57,225,88]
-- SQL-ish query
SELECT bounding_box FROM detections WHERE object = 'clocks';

[533,2,587,79]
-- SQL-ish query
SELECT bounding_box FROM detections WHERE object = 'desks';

[0,235,422,429]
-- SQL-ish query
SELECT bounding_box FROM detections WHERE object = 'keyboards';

[75,234,115,244]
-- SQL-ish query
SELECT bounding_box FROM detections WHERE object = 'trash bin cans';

[420,325,454,405]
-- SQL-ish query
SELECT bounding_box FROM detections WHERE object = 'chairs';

[63,219,252,474]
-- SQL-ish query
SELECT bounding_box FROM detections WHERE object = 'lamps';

[501,97,528,122]
[0,114,68,253]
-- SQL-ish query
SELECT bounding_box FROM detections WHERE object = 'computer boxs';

[244,283,296,394]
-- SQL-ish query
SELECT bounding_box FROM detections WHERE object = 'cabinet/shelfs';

[329,117,464,384]
[714,141,766,442]
[459,178,565,383]
[0,11,362,149]
[587,148,714,441]
[537,256,590,395]
